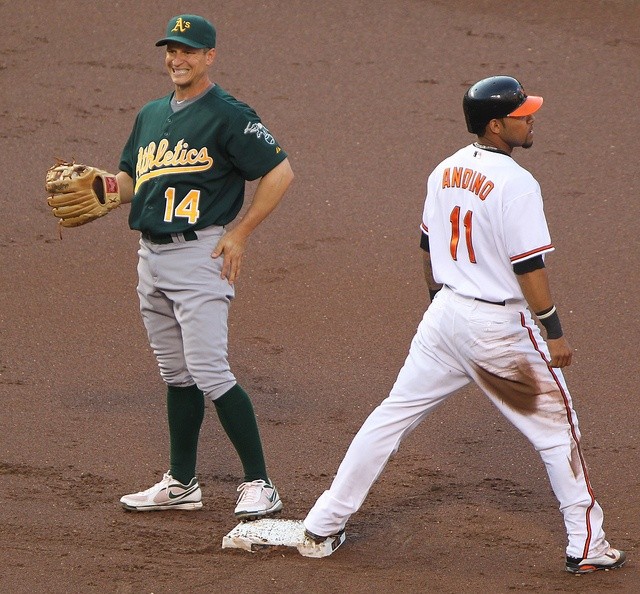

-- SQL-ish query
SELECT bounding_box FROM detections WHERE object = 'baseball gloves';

[45,158,120,240]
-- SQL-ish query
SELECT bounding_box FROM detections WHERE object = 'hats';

[156,13,218,50]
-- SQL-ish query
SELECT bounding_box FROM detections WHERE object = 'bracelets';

[536,304,565,340]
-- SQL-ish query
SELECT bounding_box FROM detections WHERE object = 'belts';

[471,298,507,306]
[140,232,197,245]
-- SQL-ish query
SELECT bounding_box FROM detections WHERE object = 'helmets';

[463,76,544,133]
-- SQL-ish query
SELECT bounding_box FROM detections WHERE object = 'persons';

[45,13,296,519]
[304,80,627,575]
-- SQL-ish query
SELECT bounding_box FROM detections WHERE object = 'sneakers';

[566,547,627,574]
[233,478,282,517]
[120,470,204,513]
[301,528,345,550]
